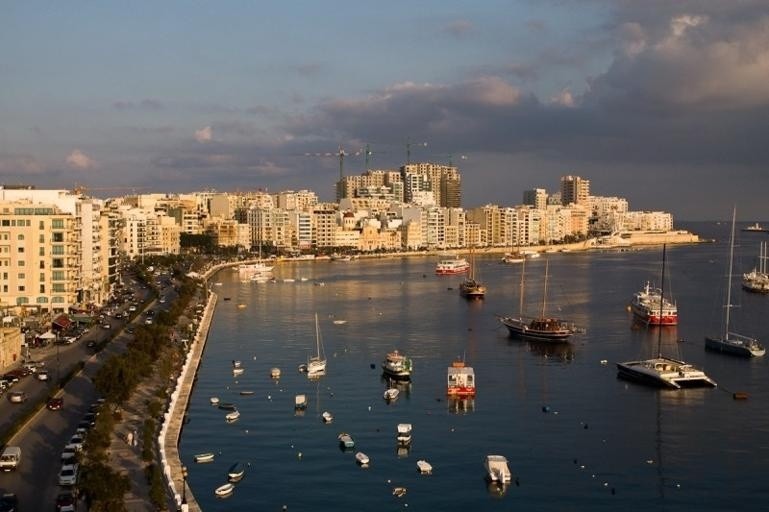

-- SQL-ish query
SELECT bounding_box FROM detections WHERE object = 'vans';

[0,446,22,473]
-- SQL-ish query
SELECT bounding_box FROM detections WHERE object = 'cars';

[1,492,19,512]
[0,358,49,402]
[145,310,155,325]
[96,287,140,334]
[57,398,106,511]
[48,399,64,413]
[63,325,87,345]
[86,340,93,346]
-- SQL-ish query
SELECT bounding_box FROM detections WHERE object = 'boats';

[741,221,768,235]
[237,264,273,272]
[447,361,476,393]
[193,360,433,498]
[500,248,570,263]
[436,257,470,275]
[632,282,678,326]
[483,454,511,482]
[333,320,348,325]
[382,350,412,378]
[240,391,256,395]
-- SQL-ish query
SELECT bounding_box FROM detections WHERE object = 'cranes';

[405,142,429,165]
[293,138,376,179]
[447,153,467,167]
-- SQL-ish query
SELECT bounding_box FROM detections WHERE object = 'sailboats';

[704,207,768,357]
[500,256,586,341]
[742,240,769,292]
[460,245,488,296]
[615,243,719,389]
[306,312,327,377]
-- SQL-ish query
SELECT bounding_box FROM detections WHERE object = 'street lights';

[181,467,187,504]
